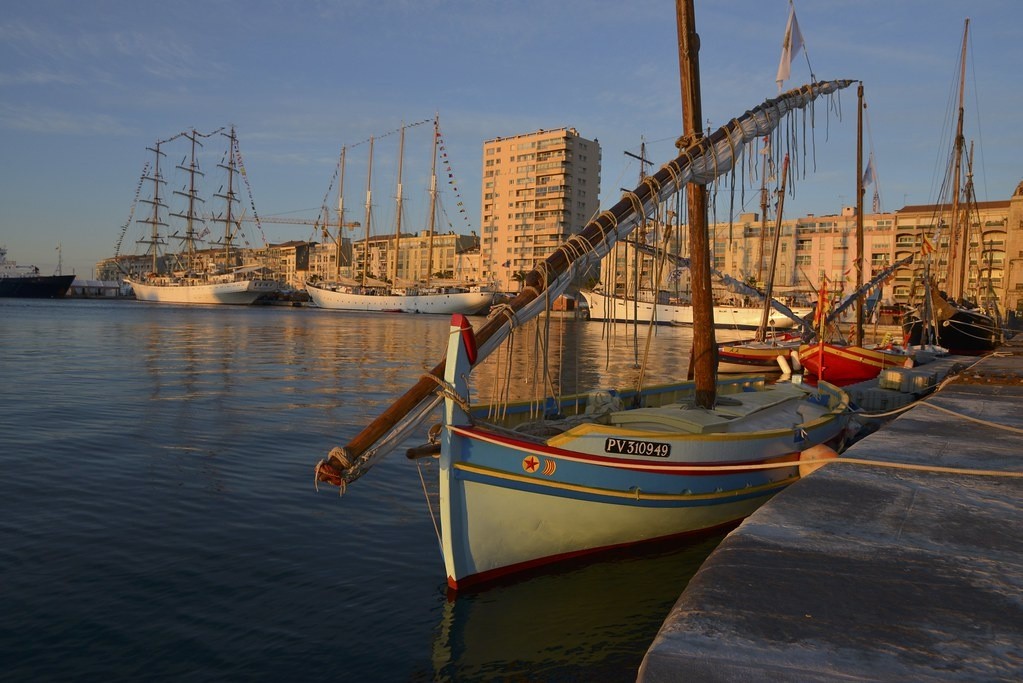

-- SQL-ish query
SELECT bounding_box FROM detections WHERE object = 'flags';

[861,158,875,188]
[921,229,936,257]
[775,4,804,90]
[812,271,830,330]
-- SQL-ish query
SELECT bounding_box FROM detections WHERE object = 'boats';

[0,246,76,300]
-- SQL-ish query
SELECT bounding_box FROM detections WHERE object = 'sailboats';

[302,113,500,314]
[579,141,815,330]
[799,79,950,382]
[901,16,1007,355]
[114,124,277,305]
[719,150,820,373]
[440,1,849,591]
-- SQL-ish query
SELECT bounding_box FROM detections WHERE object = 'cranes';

[197,206,361,245]
